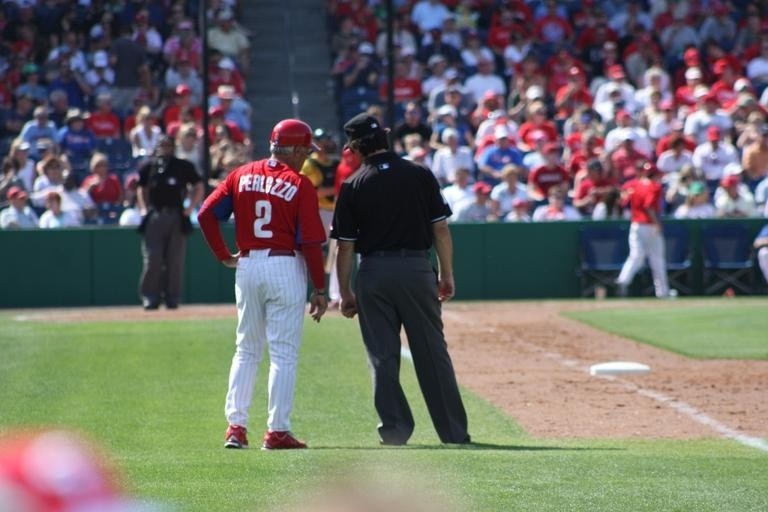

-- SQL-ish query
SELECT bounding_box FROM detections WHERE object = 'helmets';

[271,120,323,153]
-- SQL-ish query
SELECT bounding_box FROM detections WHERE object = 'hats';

[344,113,382,143]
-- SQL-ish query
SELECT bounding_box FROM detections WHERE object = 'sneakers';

[225,426,251,447]
[263,431,305,449]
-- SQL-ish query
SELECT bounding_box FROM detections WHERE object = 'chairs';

[330,0,767,296]
[0,0,251,232]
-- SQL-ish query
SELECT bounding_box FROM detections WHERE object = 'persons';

[133,134,206,309]
[328,0,767,222]
[328,112,471,446]
[612,162,677,298]
[299,129,340,302]
[322,144,361,311]
[198,119,328,451]
[0,2,255,227]
[753,225,768,281]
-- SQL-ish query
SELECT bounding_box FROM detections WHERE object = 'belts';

[363,248,431,258]
[237,248,296,256]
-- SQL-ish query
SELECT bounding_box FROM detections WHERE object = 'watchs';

[313,288,327,296]
